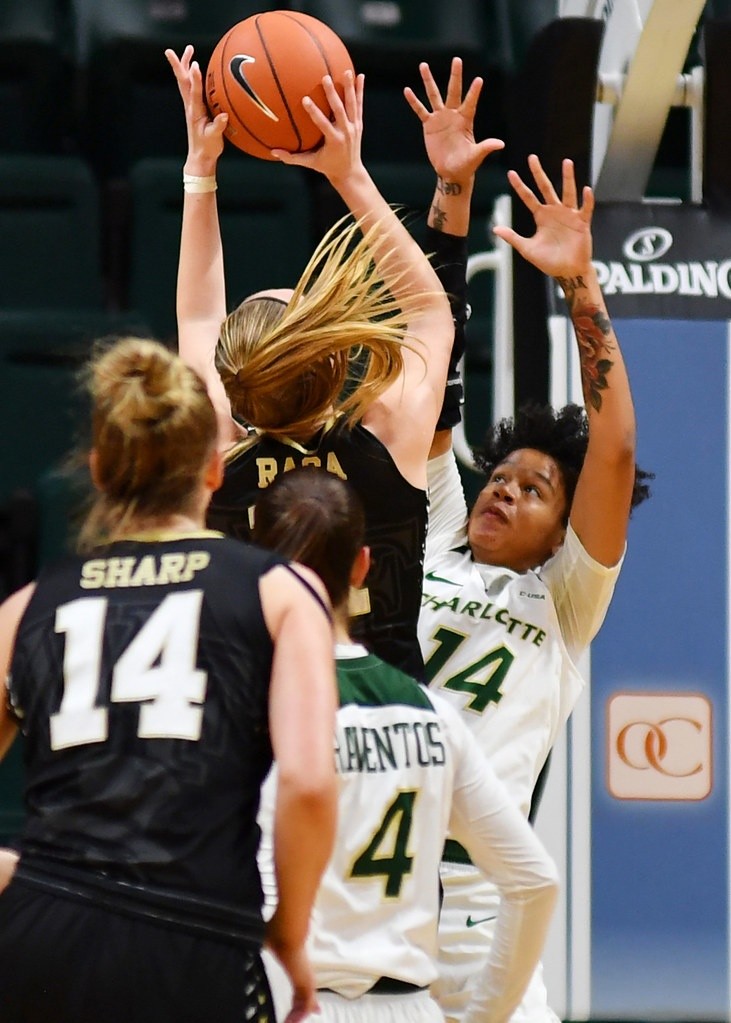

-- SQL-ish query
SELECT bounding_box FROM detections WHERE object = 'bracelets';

[183,175,218,193]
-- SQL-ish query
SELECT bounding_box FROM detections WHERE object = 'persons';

[0,45,656,1023]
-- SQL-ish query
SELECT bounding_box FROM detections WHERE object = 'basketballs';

[204,9,356,165]
